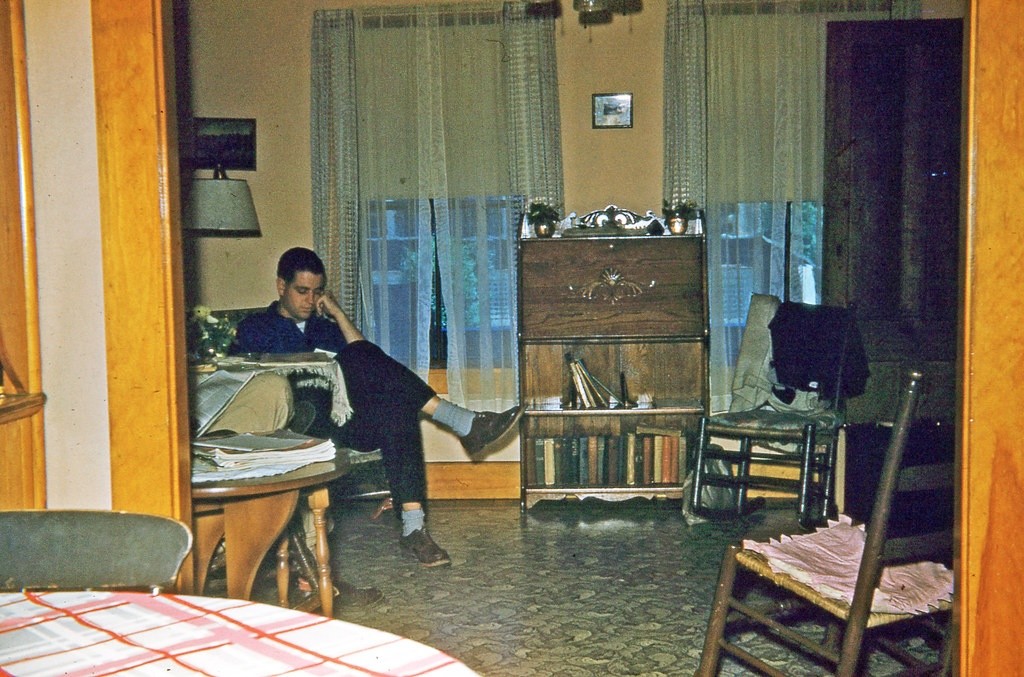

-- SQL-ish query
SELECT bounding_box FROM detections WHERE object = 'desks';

[191,434,351,618]
[0,581,482,677]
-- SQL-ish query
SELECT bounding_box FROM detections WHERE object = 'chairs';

[1,508,193,590]
[214,302,385,465]
[691,292,857,521]
[692,369,956,677]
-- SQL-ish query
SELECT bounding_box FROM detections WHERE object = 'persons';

[226,247,527,567]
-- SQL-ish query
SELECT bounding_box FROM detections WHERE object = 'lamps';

[575,1,609,30]
[183,177,262,236]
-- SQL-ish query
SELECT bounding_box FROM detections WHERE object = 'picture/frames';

[591,91,634,129]
[193,115,259,171]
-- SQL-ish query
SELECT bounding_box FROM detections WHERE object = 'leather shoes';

[400,528,450,568]
[460,403,530,459]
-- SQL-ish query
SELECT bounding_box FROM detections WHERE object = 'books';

[635,422,682,435]
[525,433,691,484]
[560,354,622,408]
[190,431,336,473]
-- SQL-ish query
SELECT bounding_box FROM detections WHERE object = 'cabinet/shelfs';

[513,199,711,516]
